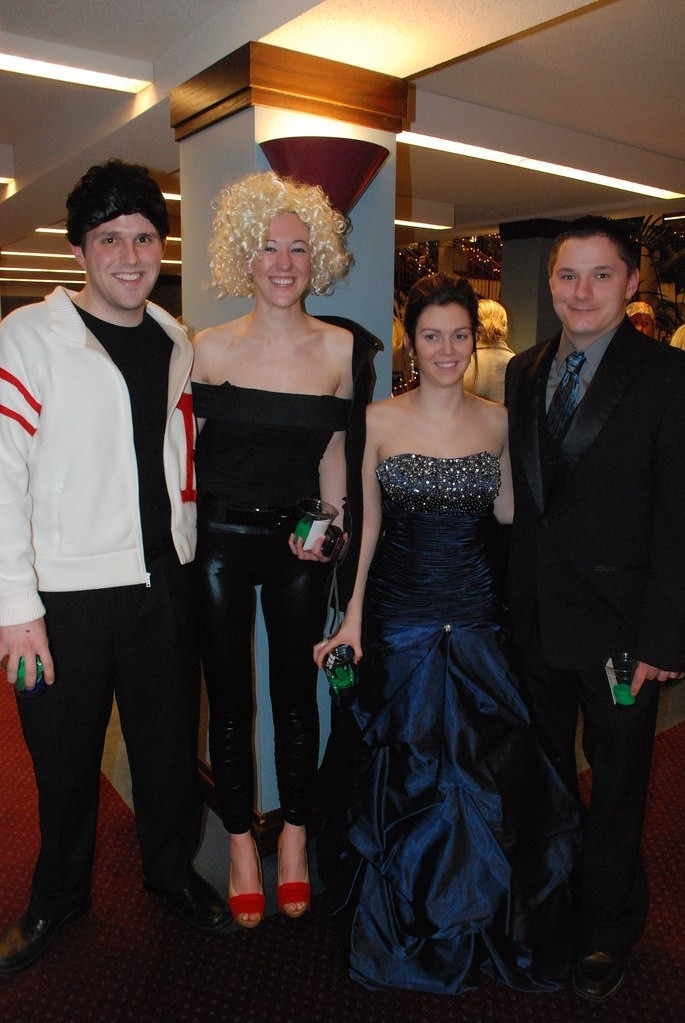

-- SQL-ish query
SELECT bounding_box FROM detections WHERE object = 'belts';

[203,507,294,528]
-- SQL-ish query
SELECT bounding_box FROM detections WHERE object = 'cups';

[290,497,339,552]
[603,649,639,705]
[322,646,355,695]
[1,655,44,691]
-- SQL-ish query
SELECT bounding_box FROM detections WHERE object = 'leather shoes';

[143,874,232,931]
[0,893,91,974]
[569,940,626,1002]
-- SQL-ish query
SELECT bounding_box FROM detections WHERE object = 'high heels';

[227,837,266,929]
[277,844,311,918]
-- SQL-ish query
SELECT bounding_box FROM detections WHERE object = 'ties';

[543,350,586,471]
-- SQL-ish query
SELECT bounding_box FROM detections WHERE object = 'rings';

[676,672,683,679]
[318,642,327,648]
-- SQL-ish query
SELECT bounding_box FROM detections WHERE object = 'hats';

[626,302,655,322]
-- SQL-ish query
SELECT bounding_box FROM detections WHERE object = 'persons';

[0,159,232,972]
[505,214,685,1001]
[391,300,413,397]
[189,171,383,929]
[461,298,517,404]
[626,302,656,338]
[312,272,590,998]
[668,323,685,351]
